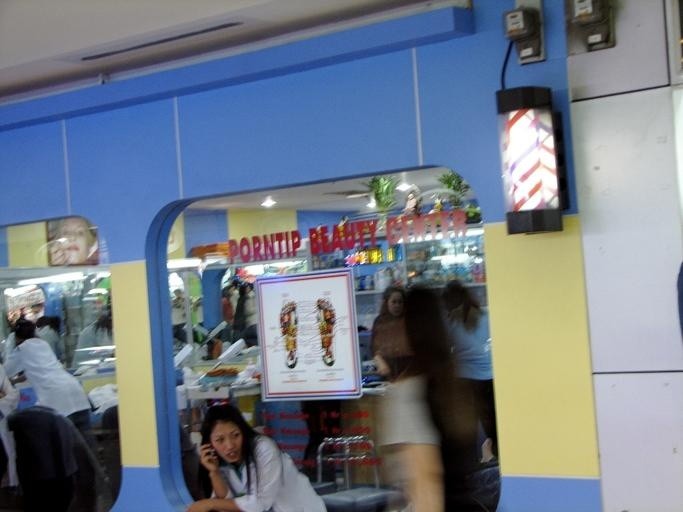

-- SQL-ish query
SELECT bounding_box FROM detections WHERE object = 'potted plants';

[436,172,469,212]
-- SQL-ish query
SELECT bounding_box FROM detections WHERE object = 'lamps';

[495,84,565,236]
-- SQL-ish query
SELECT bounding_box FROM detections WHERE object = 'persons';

[442,278,498,465]
[184,401,327,512]
[70,313,112,368]
[49,217,98,266]
[2,288,79,385]
[168,279,257,329]
[368,282,405,381]
[0,362,20,488]
[2,319,93,446]
[371,284,484,511]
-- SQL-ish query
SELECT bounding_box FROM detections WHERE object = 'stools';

[315,435,380,490]
[321,485,400,512]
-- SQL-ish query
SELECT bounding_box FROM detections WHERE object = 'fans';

[344,185,378,208]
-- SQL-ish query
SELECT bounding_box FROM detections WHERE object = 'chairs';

[5,404,98,512]
